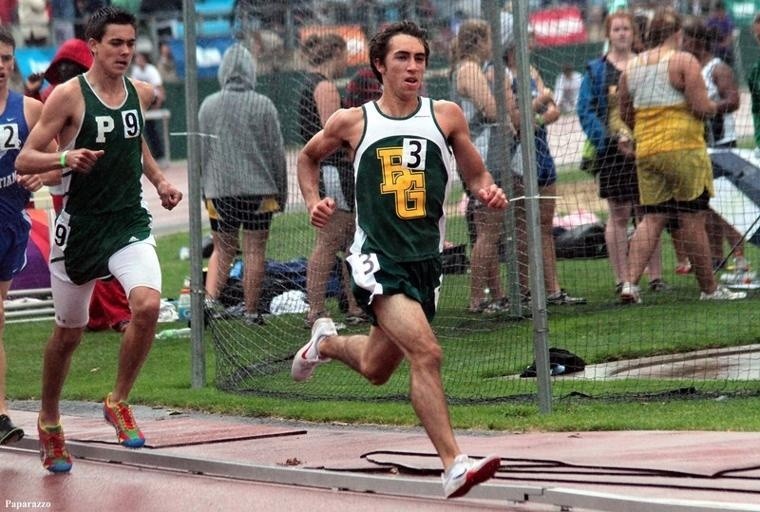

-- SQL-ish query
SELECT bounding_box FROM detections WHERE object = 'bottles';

[176,287,191,320]
[734,250,752,285]
[154,327,192,341]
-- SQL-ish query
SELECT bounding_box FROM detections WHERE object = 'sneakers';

[675,260,694,274]
[303,307,379,332]
[102,392,146,449]
[0,414,25,446]
[614,280,648,306]
[699,256,760,302]
[439,453,502,500]
[464,286,587,320]
[651,282,673,295]
[36,409,73,473]
[186,297,270,336]
[291,316,339,383]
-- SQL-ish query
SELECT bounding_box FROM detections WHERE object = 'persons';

[0,29,57,448]
[15,6,184,472]
[0,1,760,335]
[290,19,508,499]
